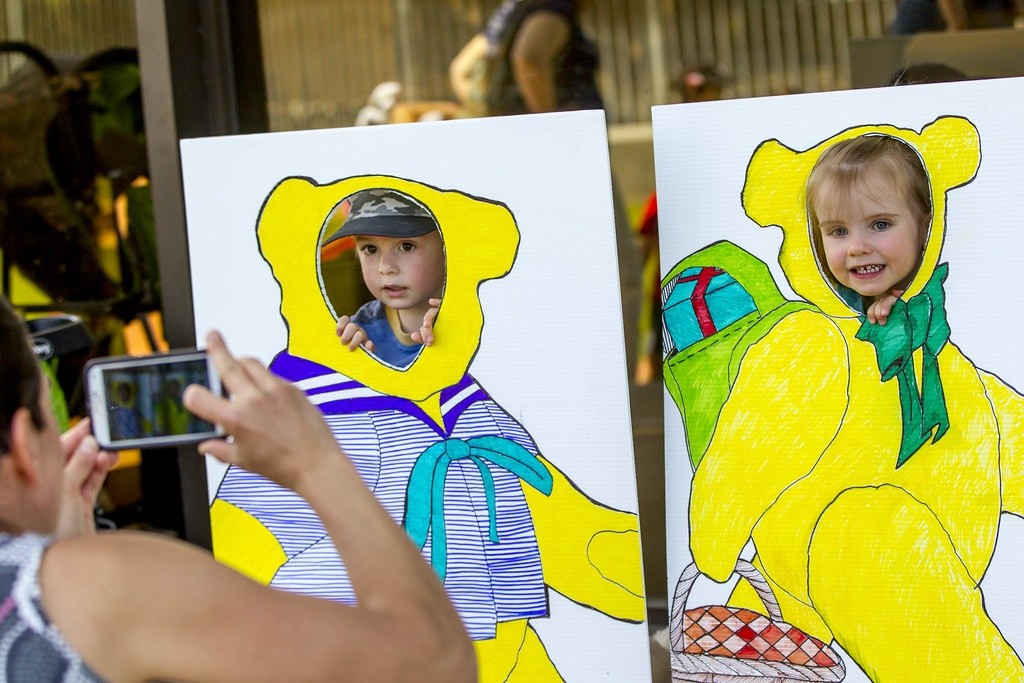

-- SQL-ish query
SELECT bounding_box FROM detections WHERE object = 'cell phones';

[81,346,231,451]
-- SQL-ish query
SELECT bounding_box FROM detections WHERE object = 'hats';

[322,190,436,247]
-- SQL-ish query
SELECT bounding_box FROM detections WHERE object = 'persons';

[0,46,139,302]
[805,137,932,327]
[891,0,1024,82]
[321,189,443,369]
[109,380,141,439]
[0,294,480,683]
[154,379,189,434]
[485,1,604,117]
